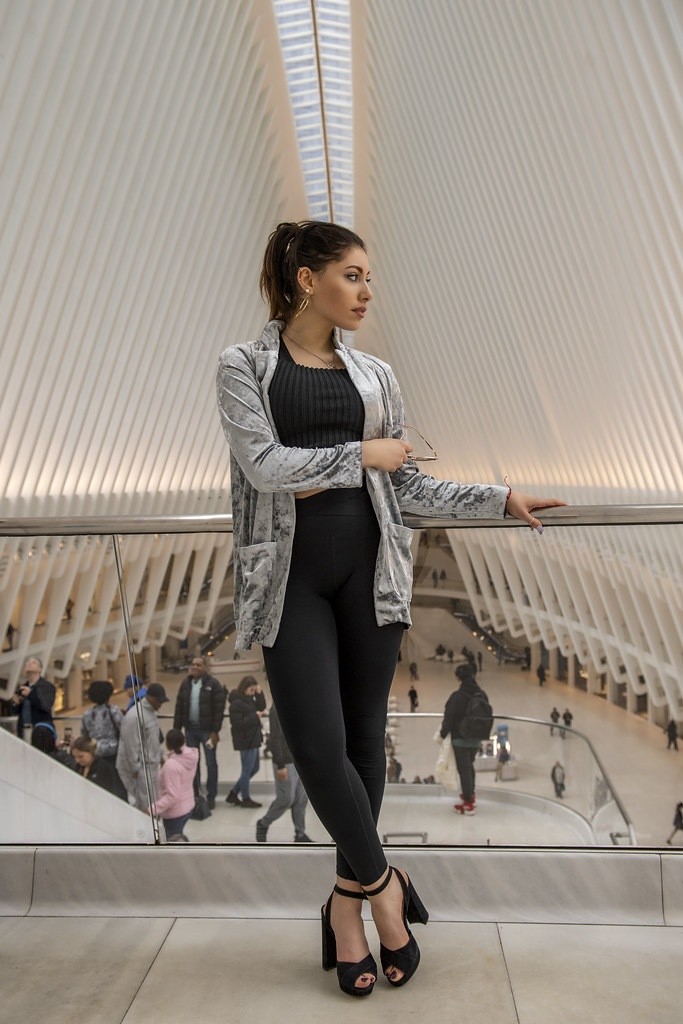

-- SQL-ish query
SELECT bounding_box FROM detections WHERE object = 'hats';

[123,674,143,690]
[88,681,113,702]
[148,683,171,703]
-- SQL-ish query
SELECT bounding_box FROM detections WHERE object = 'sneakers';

[454,792,477,815]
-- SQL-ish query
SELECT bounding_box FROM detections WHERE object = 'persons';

[398,651,418,712]
[66,599,72,619]
[663,719,678,749]
[440,665,488,817]
[6,624,13,648]
[550,706,573,738]
[215,220,568,998]
[667,802,683,845]
[423,533,440,545]
[524,646,545,686]
[551,761,565,798]
[495,748,509,781]
[1,655,312,842]
[432,568,447,588]
[385,732,436,784]
[435,644,482,672]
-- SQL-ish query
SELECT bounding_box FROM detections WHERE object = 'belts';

[22,726,33,729]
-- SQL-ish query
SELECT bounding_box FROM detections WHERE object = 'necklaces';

[284,332,335,369]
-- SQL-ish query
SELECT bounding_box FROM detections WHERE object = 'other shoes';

[255,818,267,843]
[243,798,261,808]
[227,792,244,806]
[295,834,312,843]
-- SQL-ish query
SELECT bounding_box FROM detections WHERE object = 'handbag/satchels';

[189,795,212,820]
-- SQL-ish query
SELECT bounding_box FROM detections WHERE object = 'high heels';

[361,864,429,985]
[319,881,382,1006]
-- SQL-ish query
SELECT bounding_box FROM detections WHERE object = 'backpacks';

[456,690,493,743]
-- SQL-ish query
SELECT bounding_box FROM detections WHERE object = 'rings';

[529,518,534,524]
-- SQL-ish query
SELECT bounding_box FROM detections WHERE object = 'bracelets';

[503,475,512,518]
